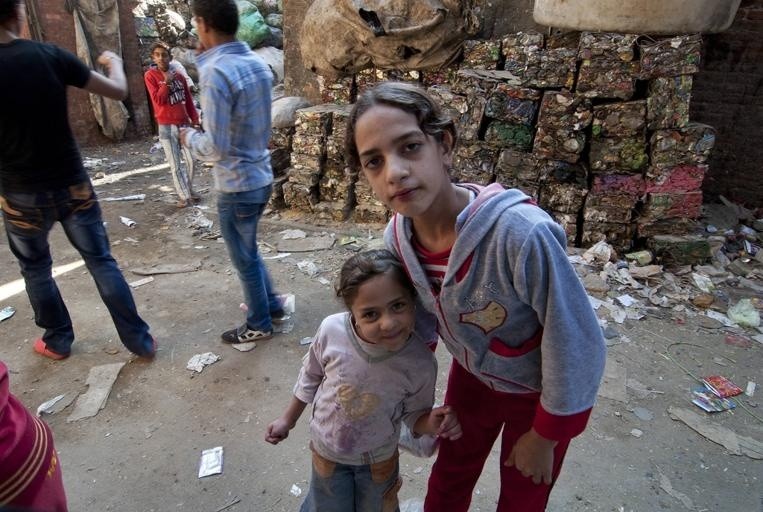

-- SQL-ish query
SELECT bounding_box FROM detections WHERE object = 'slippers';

[34,337,70,360]
[151,335,158,355]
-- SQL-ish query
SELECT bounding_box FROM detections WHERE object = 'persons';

[0,360,67,512]
[0,0,156,359]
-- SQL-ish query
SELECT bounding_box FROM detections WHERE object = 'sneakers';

[221,316,285,344]
